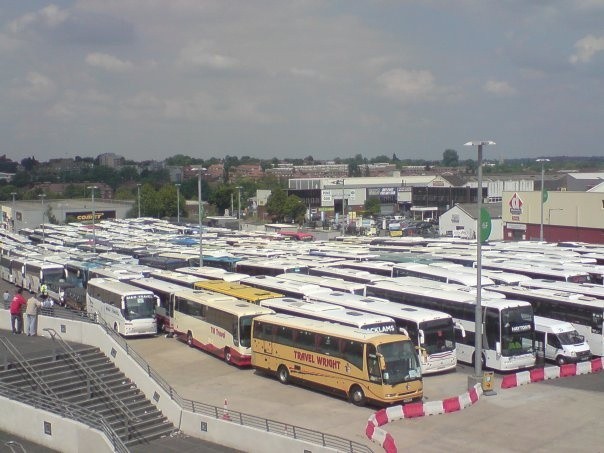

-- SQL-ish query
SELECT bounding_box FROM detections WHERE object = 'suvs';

[64,287,86,312]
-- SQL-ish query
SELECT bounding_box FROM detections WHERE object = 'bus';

[276,270,367,299]
[25,260,65,299]
[331,292,457,378]
[484,240,604,282]
[0,254,26,283]
[198,226,490,280]
[519,279,604,302]
[94,216,197,277]
[86,277,160,338]
[483,282,603,357]
[37,210,92,283]
[0,226,36,257]
[47,282,76,307]
[242,276,334,302]
[129,274,198,335]
[251,313,424,408]
[533,314,592,366]
[261,298,396,340]
[171,288,276,368]
[12,257,44,290]
[194,280,285,304]
[369,279,537,373]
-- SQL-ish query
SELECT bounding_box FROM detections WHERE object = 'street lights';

[87,185,100,253]
[465,141,497,394]
[192,168,208,268]
[536,157,551,242]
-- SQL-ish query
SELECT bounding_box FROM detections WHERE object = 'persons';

[49,297,55,316]
[25,293,42,337]
[9,288,27,334]
[2,290,9,310]
[44,297,50,314]
[42,299,46,312]
